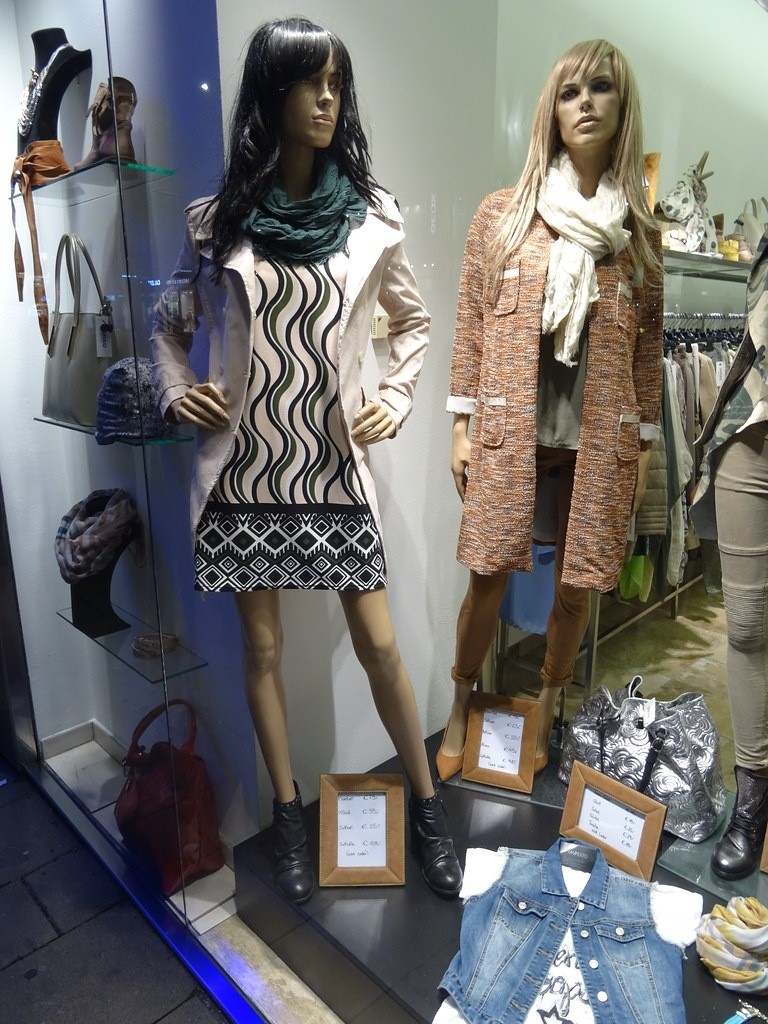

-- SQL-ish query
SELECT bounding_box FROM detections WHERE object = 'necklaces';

[18,43,73,137]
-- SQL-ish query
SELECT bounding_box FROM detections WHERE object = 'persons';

[437,39,666,777]
[148,21,462,892]
[692,232,768,880]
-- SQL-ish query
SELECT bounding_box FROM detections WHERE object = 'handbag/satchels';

[94,360,167,446]
[114,699,226,899]
[558,671,727,843]
[40,232,118,428]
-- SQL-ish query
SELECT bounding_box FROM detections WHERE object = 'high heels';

[436,707,468,782]
[534,713,555,773]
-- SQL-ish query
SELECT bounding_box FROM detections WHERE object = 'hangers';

[662,312,746,357]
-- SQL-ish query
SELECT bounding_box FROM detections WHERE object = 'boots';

[72,78,137,171]
[408,788,464,896]
[267,779,313,904]
[711,764,768,880]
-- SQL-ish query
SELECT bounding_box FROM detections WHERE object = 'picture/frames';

[558,760,667,882]
[461,691,542,794]
[318,773,405,887]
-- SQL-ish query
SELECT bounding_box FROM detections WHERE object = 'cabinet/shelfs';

[10,160,208,684]
